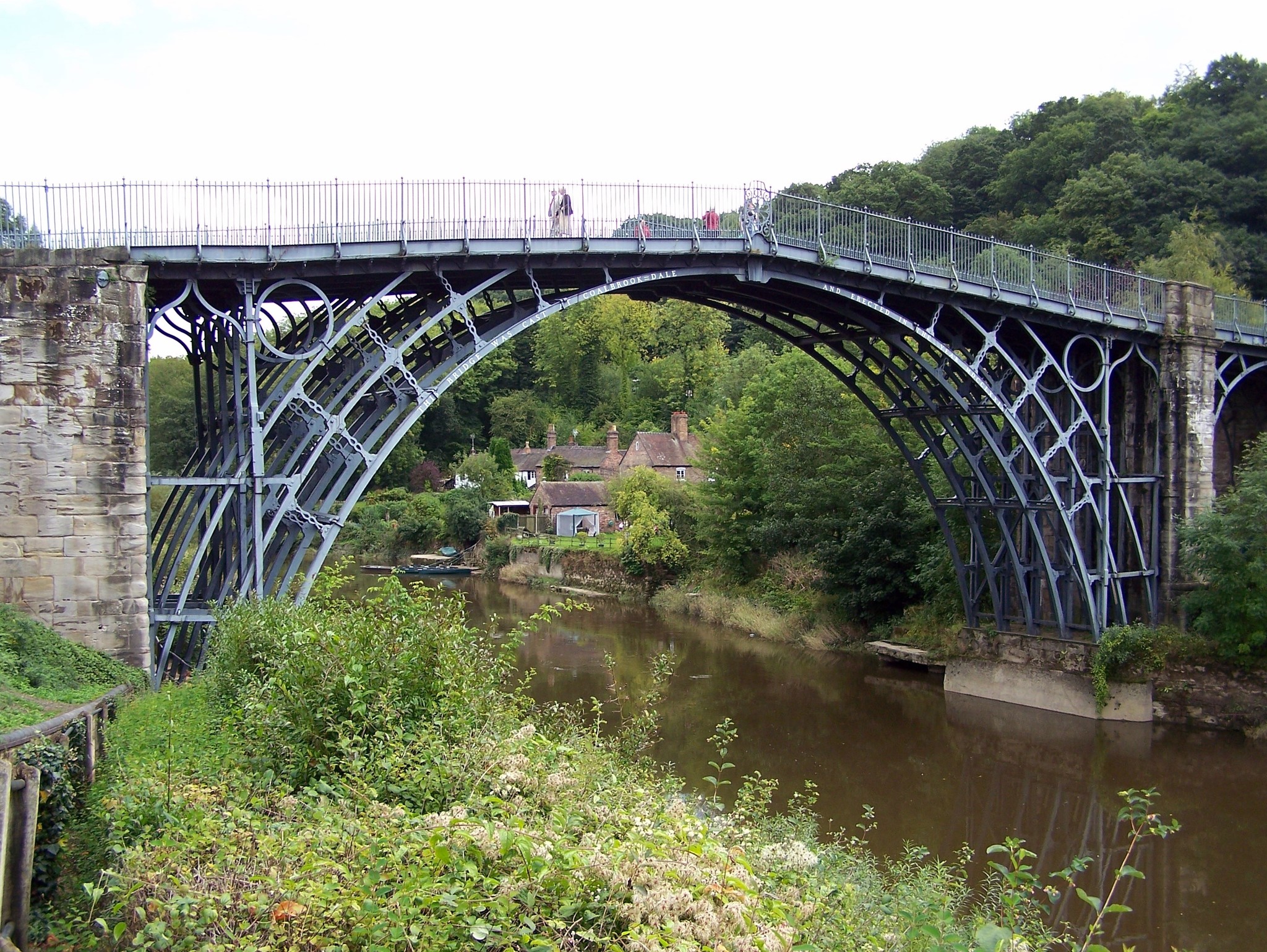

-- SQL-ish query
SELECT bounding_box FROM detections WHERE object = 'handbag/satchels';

[549,209,552,216]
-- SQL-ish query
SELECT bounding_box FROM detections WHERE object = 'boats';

[360,564,482,574]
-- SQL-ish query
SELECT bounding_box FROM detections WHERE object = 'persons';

[702,209,720,238]
[739,201,757,233]
[548,188,573,237]
[619,521,623,530]
[633,219,651,238]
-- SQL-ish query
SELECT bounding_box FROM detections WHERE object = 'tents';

[556,507,599,537]
